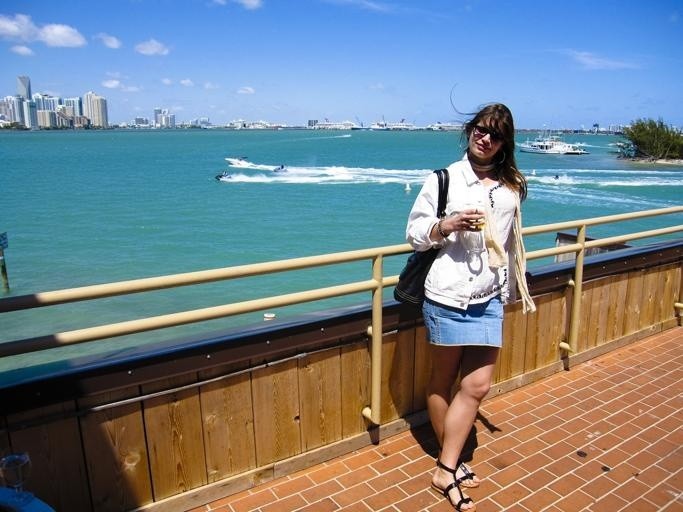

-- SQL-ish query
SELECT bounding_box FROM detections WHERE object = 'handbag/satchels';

[393,247,439,307]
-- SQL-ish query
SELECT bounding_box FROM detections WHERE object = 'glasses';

[474,124,502,141]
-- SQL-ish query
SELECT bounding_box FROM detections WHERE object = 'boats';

[223,158,252,168]
[534,135,560,141]
[519,142,588,154]
[215,174,231,181]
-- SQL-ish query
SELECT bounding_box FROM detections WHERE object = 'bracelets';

[436,219,449,239]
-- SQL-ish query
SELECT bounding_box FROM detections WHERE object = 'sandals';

[431,447,479,512]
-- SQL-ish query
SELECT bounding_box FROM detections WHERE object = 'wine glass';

[469,204,485,253]
[0,452,36,505]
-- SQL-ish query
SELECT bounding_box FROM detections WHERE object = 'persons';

[404,104,535,512]
